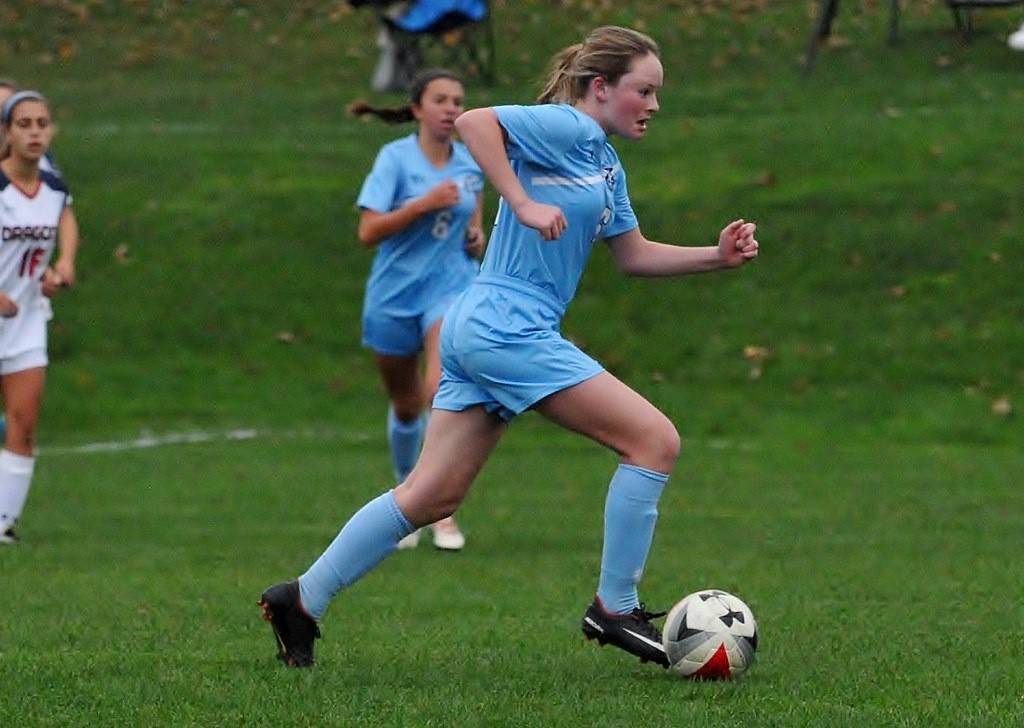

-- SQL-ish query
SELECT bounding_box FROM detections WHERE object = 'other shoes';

[399,516,466,551]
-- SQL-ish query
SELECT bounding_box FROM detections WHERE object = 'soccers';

[661,590,762,680]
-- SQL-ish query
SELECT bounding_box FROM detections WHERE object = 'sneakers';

[258,579,317,669]
[583,595,674,669]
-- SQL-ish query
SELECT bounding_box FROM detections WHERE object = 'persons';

[259,27,761,670]
[1,81,65,299]
[348,73,488,555]
[0,91,81,552]
